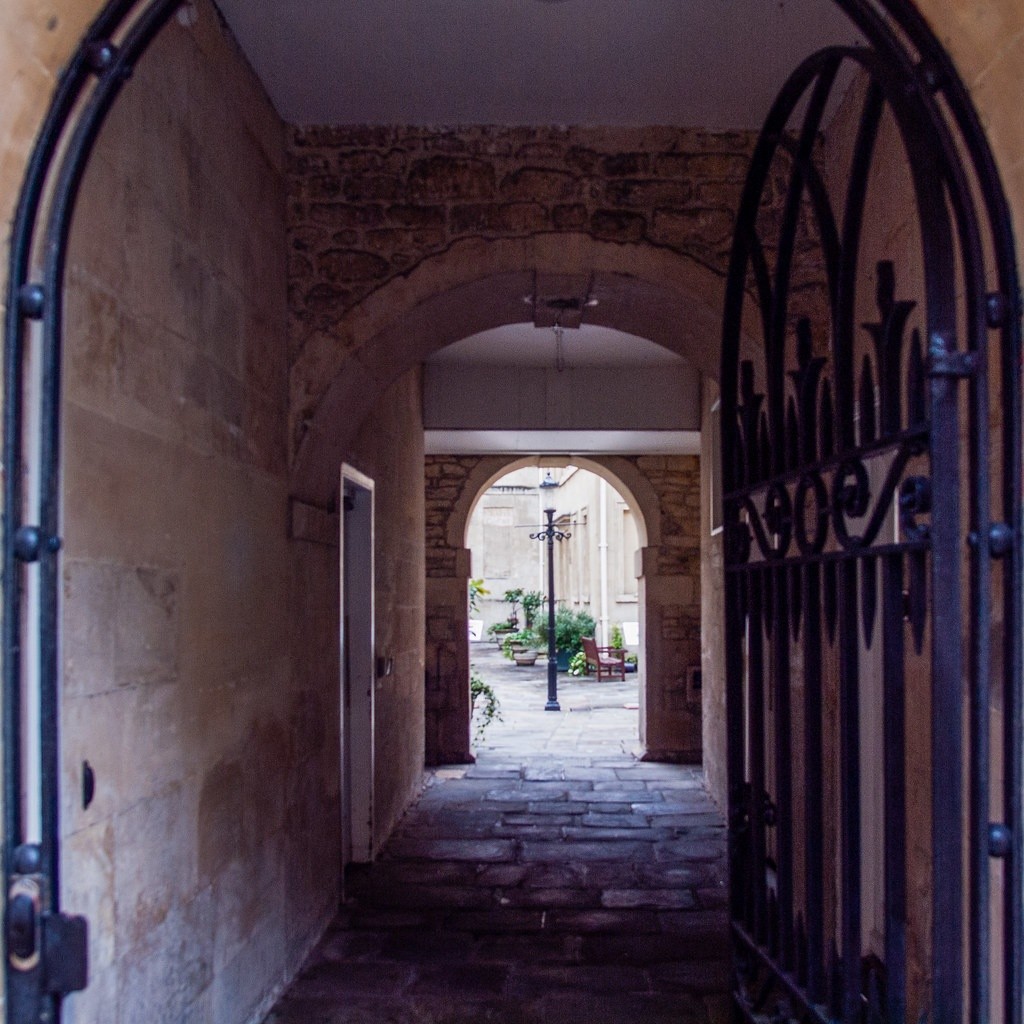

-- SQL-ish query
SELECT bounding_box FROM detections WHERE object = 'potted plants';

[503,629,548,667]
[486,620,519,650]
[533,607,598,672]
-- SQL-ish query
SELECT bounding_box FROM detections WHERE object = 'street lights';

[529,473,572,710]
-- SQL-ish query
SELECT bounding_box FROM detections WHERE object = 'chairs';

[579,635,628,683]
